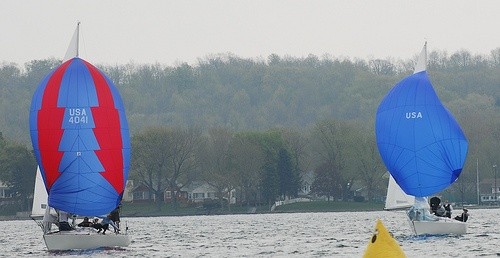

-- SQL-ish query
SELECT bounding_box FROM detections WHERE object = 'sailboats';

[28,23,132,252]
[375,42,471,237]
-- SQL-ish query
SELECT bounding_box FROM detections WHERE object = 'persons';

[77,217,92,227]
[430,196,445,216]
[455,208,469,222]
[58,210,75,231]
[442,200,454,218]
[110,206,120,234]
[96,215,120,235]
[91,217,101,227]
